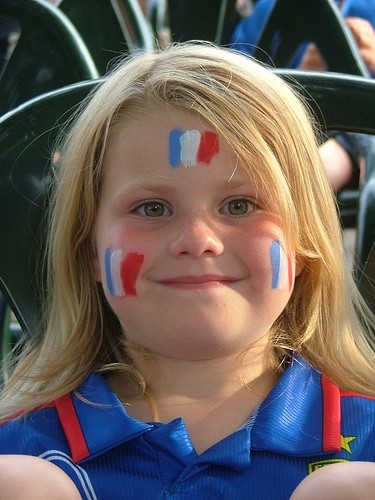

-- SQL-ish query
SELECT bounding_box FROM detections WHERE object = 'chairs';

[0,0,375,385]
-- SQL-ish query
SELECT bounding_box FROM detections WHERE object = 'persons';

[1,0,375,198]
[0,45,375,500]
